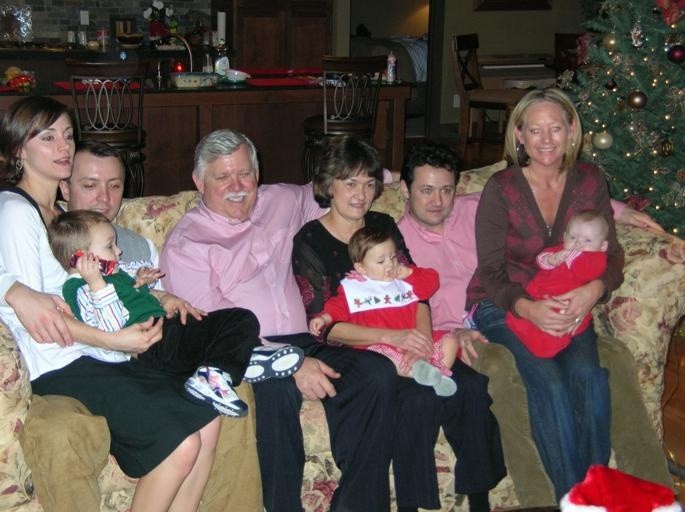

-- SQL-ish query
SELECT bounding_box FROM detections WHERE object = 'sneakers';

[409,357,458,398]
[183,364,249,418]
[241,344,304,383]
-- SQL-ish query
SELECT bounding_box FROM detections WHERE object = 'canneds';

[66,30,76,49]
[78,31,87,46]
[96,30,110,48]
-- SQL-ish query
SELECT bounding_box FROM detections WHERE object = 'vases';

[149,21,167,38]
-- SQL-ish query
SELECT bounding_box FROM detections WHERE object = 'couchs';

[2,159,685,512]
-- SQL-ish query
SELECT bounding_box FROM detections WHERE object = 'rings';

[576,318,580,324]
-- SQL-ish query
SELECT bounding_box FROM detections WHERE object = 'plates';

[116,32,144,45]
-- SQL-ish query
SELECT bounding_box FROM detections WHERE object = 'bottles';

[387,51,396,85]
[77,31,87,46]
[66,30,78,44]
[96,31,110,47]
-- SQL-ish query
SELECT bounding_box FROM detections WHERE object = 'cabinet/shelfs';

[211,0,334,69]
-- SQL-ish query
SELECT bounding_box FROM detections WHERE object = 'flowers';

[143,1,174,23]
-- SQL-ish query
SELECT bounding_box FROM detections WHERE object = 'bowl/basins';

[171,72,221,88]
[223,69,251,84]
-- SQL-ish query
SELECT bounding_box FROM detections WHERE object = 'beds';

[350,34,429,119]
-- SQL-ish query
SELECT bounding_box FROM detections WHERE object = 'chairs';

[303,54,387,183]
[452,33,531,161]
[70,75,148,198]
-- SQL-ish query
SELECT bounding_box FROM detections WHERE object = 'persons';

[160,130,401,510]
[465,89,625,505]
[507,209,609,359]
[291,128,507,511]
[396,138,666,511]
[1,97,305,512]
[309,223,461,396]
